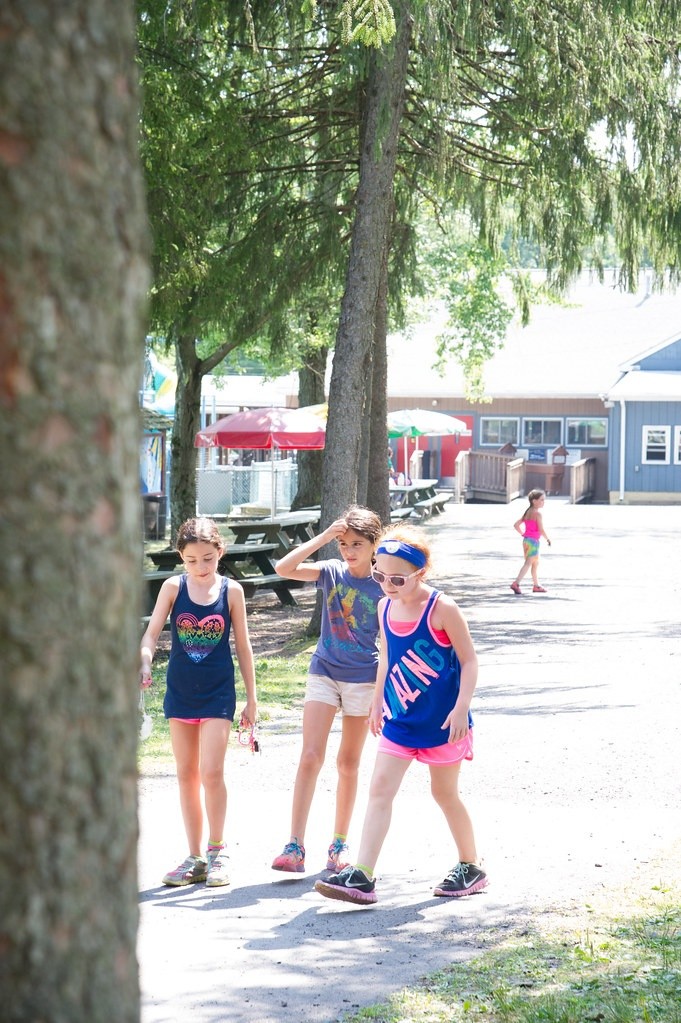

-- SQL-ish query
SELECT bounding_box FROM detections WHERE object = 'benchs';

[140,493,453,624]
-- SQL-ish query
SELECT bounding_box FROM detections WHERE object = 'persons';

[270,508,384,873]
[315,527,491,905]
[510,489,551,594]
[388,448,394,478]
[138,517,259,887]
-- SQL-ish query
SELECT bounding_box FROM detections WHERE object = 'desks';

[144,543,298,606]
[143,569,184,602]
[227,508,319,565]
[386,478,446,515]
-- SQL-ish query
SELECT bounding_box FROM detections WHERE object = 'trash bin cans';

[143,493,168,540]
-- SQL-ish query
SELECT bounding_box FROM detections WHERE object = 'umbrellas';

[300,401,328,421]
[193,406,326,523]
[387,409,466,487]
